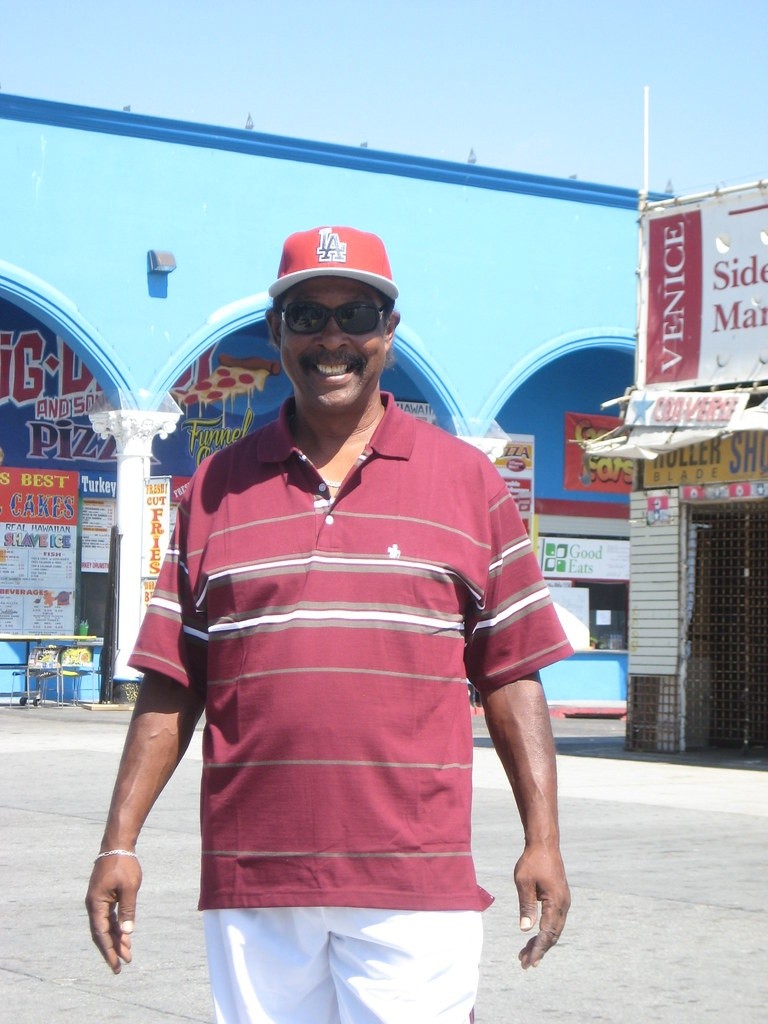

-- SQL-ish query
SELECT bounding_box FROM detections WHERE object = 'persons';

[84,227,572,1024]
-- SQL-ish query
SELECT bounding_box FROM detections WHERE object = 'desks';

[0,633,96,708]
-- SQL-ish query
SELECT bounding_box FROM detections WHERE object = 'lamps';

[148,251,177,272]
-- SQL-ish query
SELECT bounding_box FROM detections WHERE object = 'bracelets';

[96,851,137,862]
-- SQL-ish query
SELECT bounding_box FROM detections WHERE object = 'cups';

[79,624,89,636]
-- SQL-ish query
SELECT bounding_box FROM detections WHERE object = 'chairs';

[10,644,96,709]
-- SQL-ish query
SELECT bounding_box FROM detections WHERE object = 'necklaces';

[323,480,342,488]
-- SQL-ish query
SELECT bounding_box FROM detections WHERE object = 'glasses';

[277,301,384,335]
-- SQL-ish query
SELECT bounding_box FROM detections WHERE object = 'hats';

[268,226,399,301]
